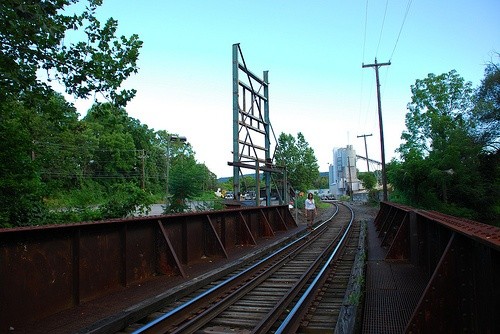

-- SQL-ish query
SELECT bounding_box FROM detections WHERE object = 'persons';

[303,192,318,232]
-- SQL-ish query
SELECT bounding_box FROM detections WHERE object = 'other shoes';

[306,228,314,231]
